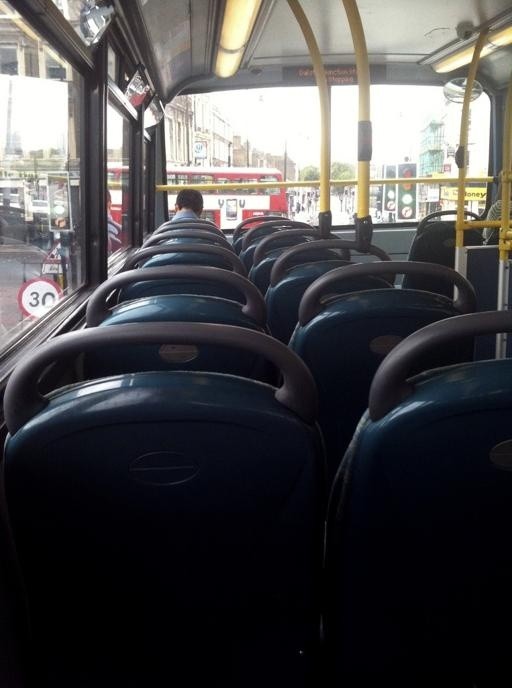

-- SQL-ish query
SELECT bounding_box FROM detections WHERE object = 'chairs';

[0,210,512,687]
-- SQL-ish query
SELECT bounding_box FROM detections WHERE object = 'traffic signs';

[17,276,63,320]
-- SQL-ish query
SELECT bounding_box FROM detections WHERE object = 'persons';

[107,191,122,238]
[481,171,512,245]
[170,189,203,223]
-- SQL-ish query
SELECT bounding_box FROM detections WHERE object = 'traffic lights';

[395,162,419,221]
[48,173,73,232]
[381,164,397,213]
[376,184,382,212]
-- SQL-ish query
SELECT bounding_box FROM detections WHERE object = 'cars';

[0,236,63,339]
[27,199,49,216]
[0,214,43,250]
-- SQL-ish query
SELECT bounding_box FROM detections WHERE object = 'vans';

[0,192,32,212]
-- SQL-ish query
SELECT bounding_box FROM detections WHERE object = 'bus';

[58,161,289,233]
